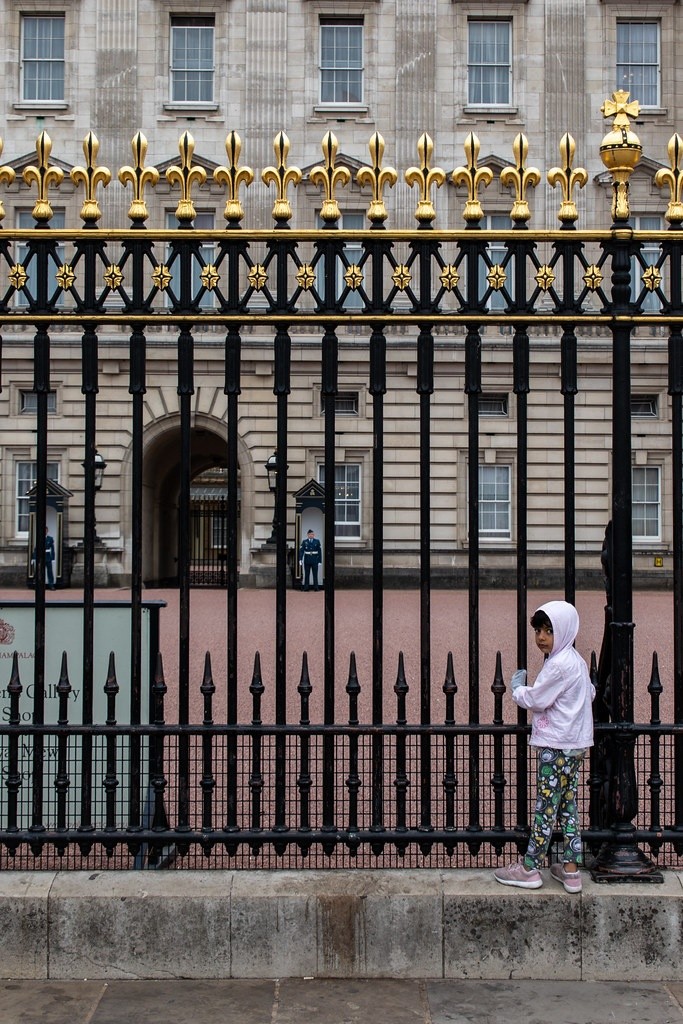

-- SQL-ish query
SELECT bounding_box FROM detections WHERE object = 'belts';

[45,549,50,552]
[304,551,318,555]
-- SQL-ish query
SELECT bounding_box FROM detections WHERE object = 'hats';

[307,529,313,534]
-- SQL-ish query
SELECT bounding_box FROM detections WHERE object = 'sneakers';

[550,860,581,892]
[492,862,543,889]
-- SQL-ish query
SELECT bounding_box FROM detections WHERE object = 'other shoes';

[50,586,54,590]
[304,587,309,591]
[313,587,318,591]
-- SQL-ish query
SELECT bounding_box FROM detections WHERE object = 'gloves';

[299,560,303,566]
[511,668,528,691]
[318,563,321,566]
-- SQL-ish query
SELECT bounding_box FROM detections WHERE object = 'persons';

[298,529,323,592]
[31,526,56,591]
[492,601,596,894]
